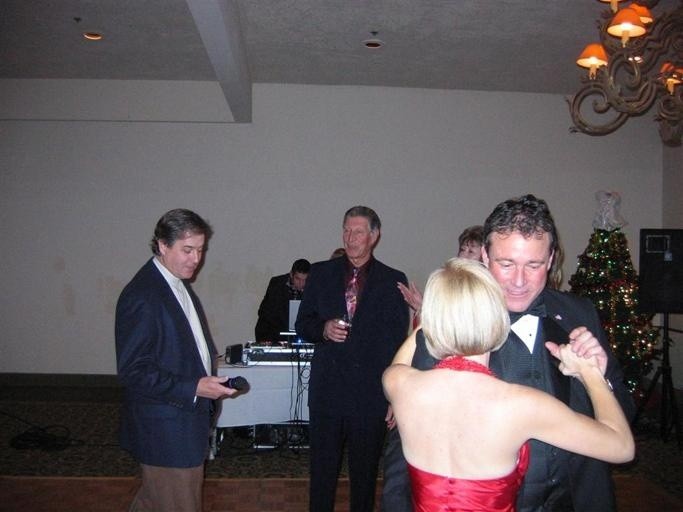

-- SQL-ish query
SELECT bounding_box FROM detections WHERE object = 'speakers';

[638,228,683,315]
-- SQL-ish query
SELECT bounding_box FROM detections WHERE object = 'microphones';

[220,376,248,390]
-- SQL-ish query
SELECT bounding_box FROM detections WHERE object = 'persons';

[382,194,636,510]
[253,260,312,345]
[114,207,241,512]
[295,206,409,512]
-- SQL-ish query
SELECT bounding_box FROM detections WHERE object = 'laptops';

[287,299,301,331]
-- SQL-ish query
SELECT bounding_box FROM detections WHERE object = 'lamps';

[562,2,681,147]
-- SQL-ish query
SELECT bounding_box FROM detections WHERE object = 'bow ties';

[509,296,548,324]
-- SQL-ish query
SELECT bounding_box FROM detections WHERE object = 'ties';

[346,267,360,326]
